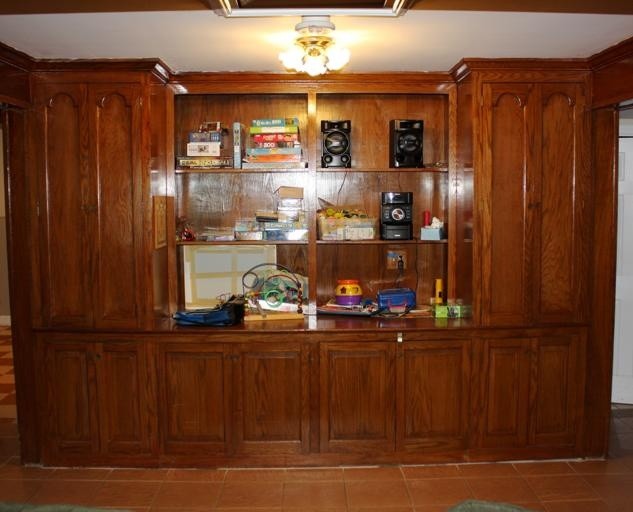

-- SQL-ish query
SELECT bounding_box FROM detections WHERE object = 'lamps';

[278,14,350,78]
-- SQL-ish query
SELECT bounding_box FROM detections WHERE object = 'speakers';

[389,120,425,168]
[321,119,351,168]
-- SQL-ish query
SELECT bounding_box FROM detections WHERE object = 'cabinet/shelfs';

[309,330,469,465]
[154,331,310,469]
[27,57,173,333]
[27,333,152,470]
[174,90,451,315]
[468,325,588,462]
[451,58,589,328]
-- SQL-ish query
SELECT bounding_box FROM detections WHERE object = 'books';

[175,116,308,168]
[197,186,308,241]
[243,297,309,321]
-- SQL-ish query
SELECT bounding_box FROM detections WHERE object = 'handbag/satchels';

[172,310,236,327]
